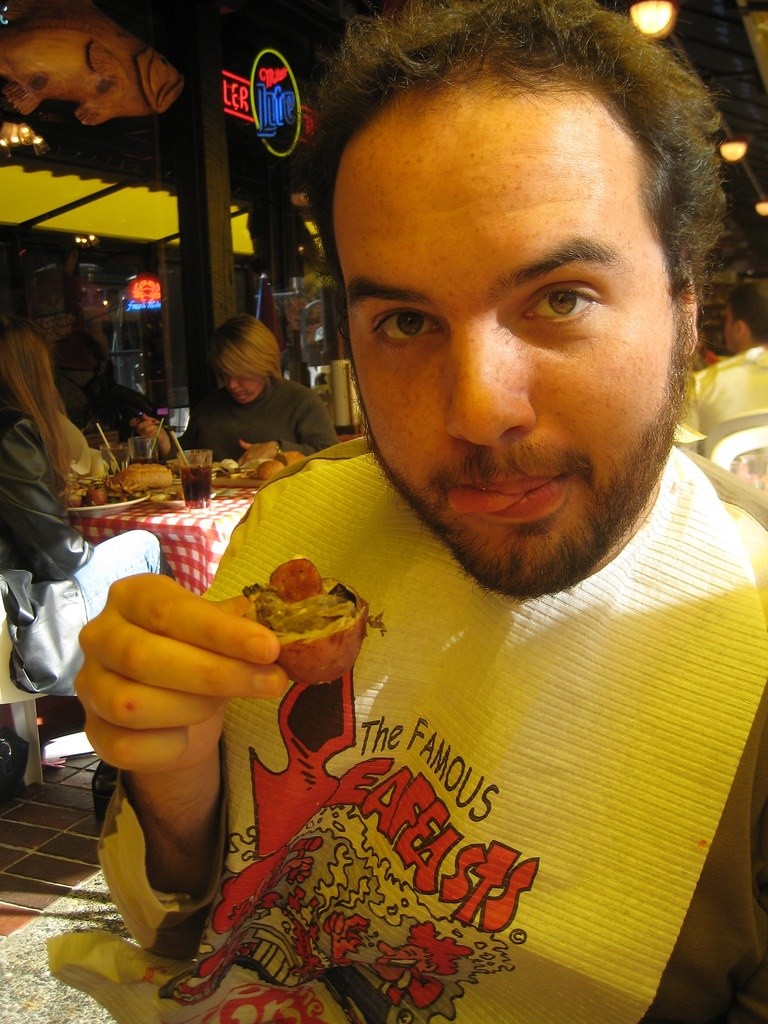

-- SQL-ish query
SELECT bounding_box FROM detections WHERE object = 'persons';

[59,282,114,417]
[74,0,767,1024]
[677,280,768,494]
[130,316,338,467]
[0,330,162,612]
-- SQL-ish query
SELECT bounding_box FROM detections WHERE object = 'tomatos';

[269,556,322,603]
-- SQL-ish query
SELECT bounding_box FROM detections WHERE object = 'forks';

[156,421,185,435]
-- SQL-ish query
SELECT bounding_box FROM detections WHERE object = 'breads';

[256,450,305,480]
[112,462,175,490]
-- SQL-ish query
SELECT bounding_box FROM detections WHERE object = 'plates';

[150,493,218,510]
[68,489,150,519]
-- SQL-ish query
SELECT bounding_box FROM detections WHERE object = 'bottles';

[299,362,334,430]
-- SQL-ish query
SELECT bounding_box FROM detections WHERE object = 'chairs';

[1,566,62,788]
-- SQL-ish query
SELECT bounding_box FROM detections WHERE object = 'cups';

[177,449,212,509]
[128,436,161,466]
[329,359,361,435]
[100,442,131,484]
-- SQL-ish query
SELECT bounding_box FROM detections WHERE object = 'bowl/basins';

[166,458,178,471]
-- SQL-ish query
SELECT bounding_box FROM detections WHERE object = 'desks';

[69,481,262,594]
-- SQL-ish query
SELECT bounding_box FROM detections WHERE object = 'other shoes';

[92,756,117,820]
